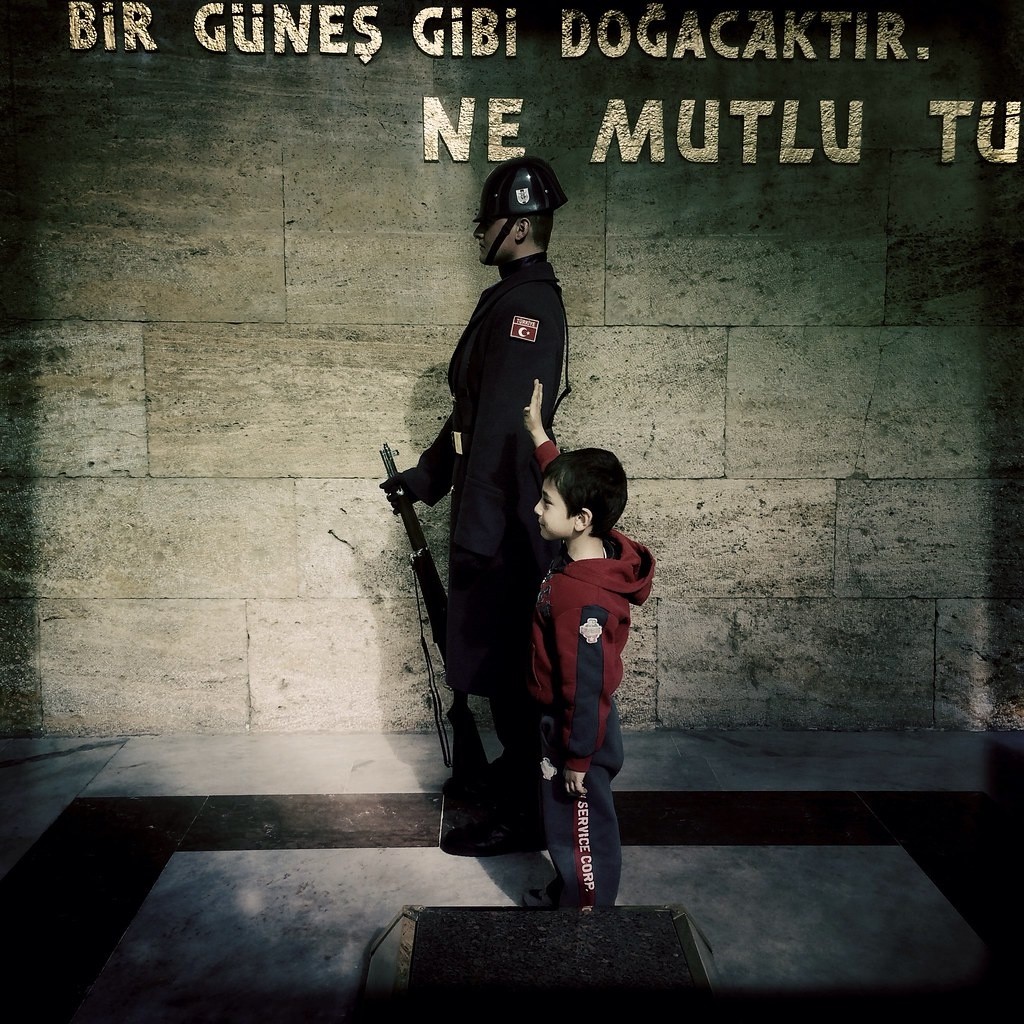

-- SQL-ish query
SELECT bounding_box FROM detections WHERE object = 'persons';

[381,155,569,857]
[522,378,656,908]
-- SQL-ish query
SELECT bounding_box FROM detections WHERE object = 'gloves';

[380,467,425,515]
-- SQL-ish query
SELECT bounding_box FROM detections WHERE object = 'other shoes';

[443,758,499,803]
[441,807,548,857]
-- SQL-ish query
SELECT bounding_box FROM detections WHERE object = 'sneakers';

[523,883,553,907]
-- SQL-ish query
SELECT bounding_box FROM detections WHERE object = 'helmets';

[472,157,568,223]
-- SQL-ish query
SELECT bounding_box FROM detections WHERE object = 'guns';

[376,443,490,785]
